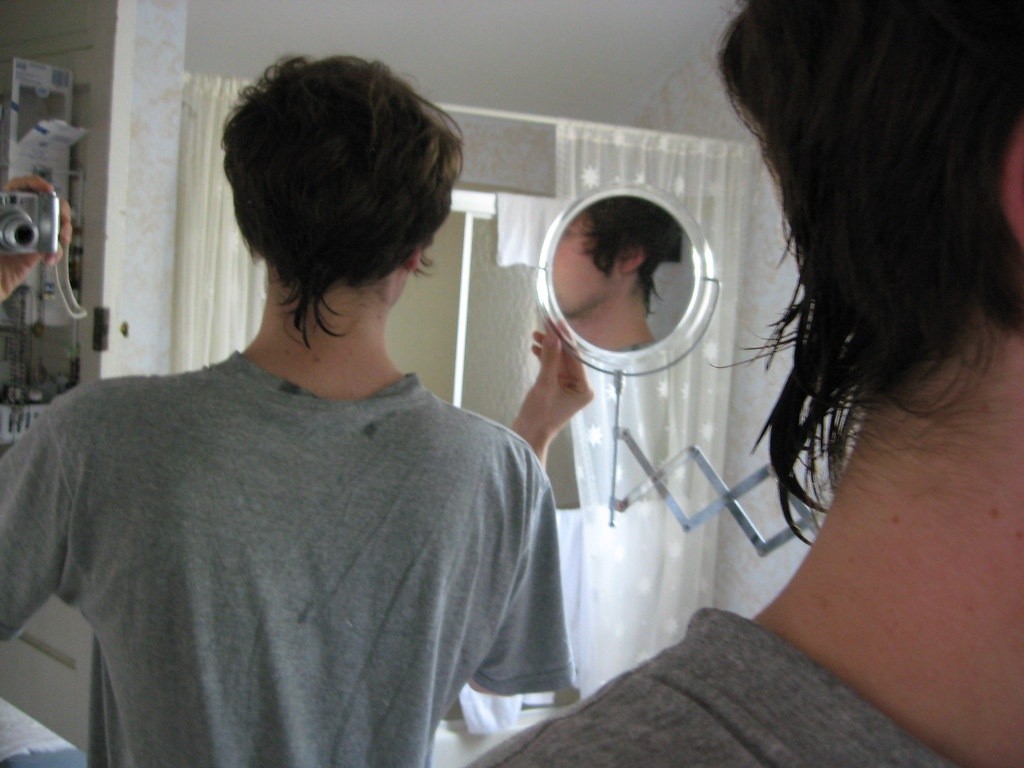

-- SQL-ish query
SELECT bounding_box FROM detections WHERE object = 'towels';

[456,507,589,735]
[494,192,565,268]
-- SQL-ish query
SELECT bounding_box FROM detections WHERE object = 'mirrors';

[534,183,722,380]
[0,0,845,767]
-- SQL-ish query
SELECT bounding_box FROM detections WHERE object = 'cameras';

[0,189,60,255]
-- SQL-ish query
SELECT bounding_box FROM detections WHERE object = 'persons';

[457,0,1024,766]
[1,50,593,768]
[552,197,683,353]
